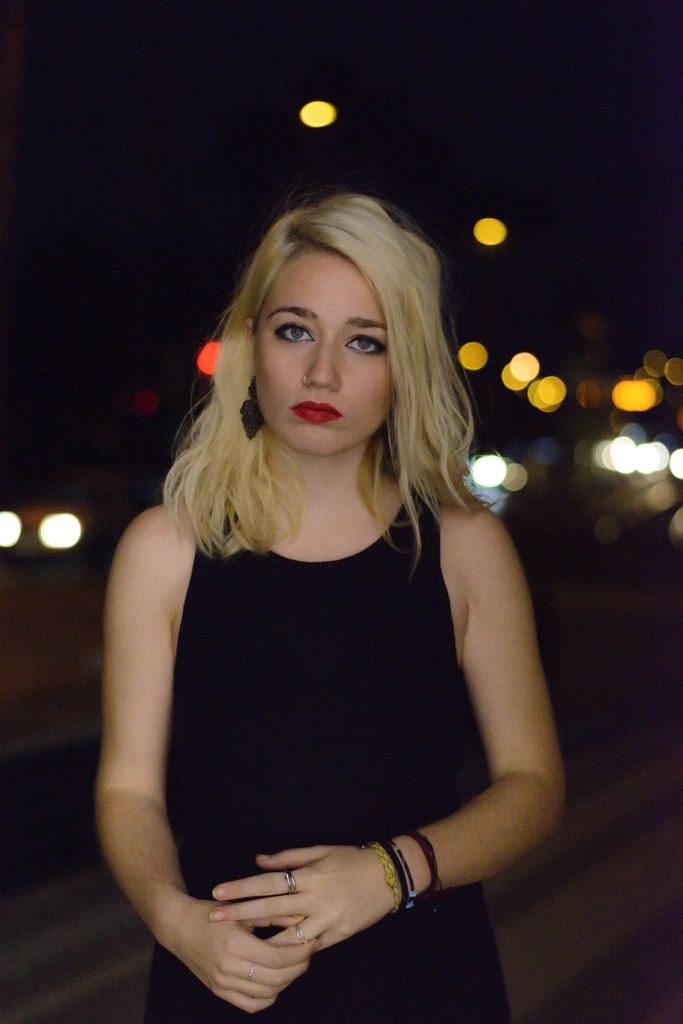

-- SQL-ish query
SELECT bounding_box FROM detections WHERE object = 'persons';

[97,190,567,1024]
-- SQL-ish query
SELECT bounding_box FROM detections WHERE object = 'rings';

[247,962,256,980]
[285,870,297,894]
[294,923,308,944]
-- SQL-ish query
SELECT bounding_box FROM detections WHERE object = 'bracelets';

[361,838,416,913]
[406,831,443,897]
